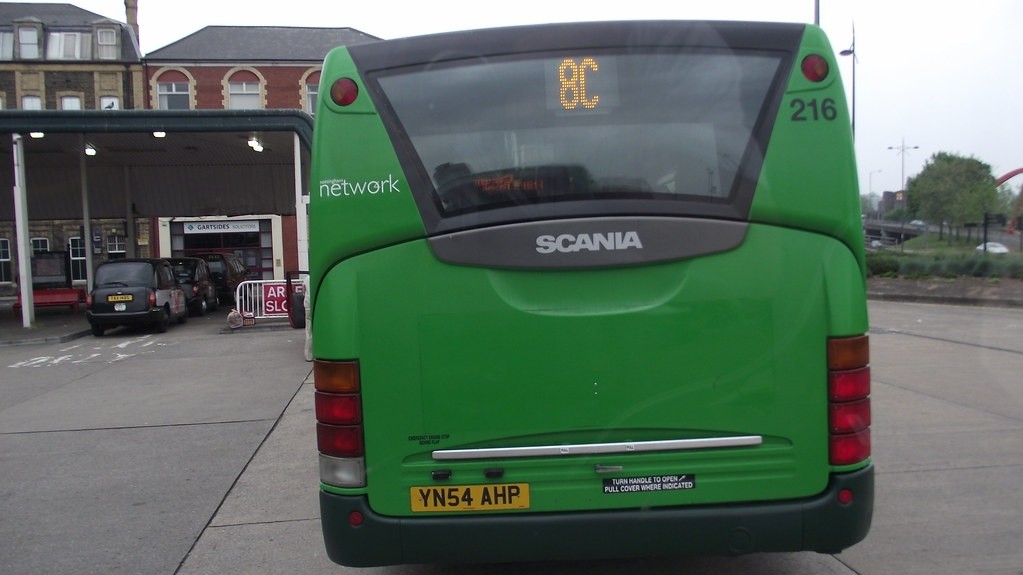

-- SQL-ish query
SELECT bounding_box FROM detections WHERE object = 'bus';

[285,18,882,569]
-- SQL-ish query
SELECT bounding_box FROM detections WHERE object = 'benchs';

[13,289,79,318]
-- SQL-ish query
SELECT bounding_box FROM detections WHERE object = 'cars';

[202,252,250,307]
[977,240,1010,255]
[84,256,189,337]
[910,219,926,227]
[160,256,221,316]
[245,263,272,283]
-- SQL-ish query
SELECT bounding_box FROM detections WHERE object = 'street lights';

[839,18,855,142]
[888,136,919,253]
